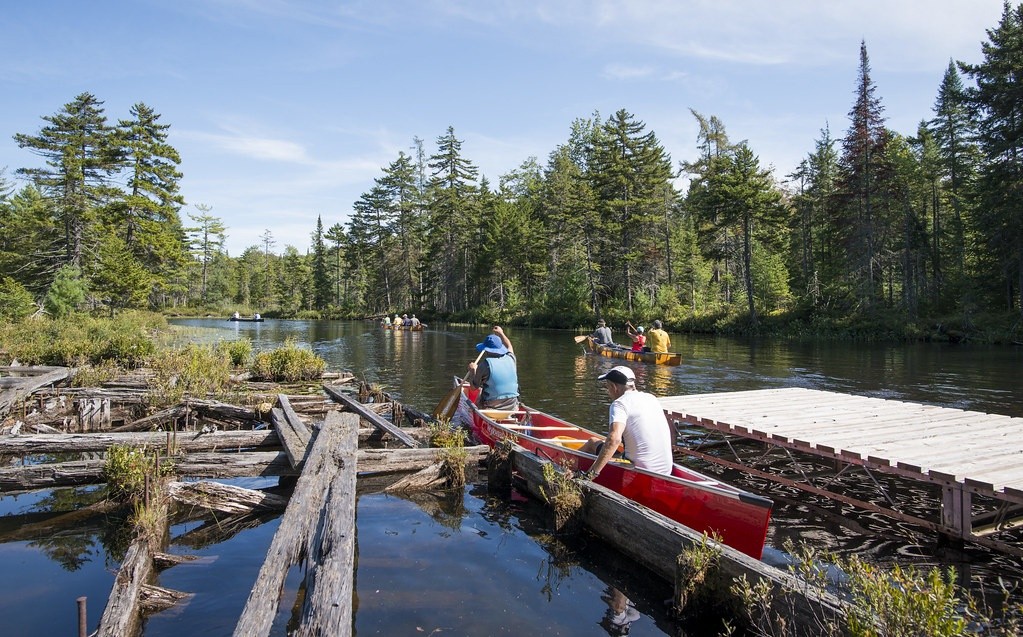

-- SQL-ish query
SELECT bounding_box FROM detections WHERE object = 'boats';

[228,317,265,322]
[380,321,427,331]
[452,375,776,562]
[587,334,683,365]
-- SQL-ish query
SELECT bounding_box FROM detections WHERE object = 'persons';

[578,366,673,481]
[381,315,390,326]
[647,320,672,353]
[469,325,521,411]
[592,319,613,345]
[401,314,411,326]
[392,314,402,325]
[625,321,646,353]
[410,314,421,326]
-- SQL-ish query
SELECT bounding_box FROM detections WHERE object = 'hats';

[652,320,662,328]
[403,314,407,317]
[598,318,606,324]
[637,326,644,333]
[597,365,636,386]
[411,314,415,317]
[476,334,508,355]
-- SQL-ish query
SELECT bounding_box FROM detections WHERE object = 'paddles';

[227,315,233,321]
[432,330,497,422]
[573,334,590,343]
[625,320,639,333]
